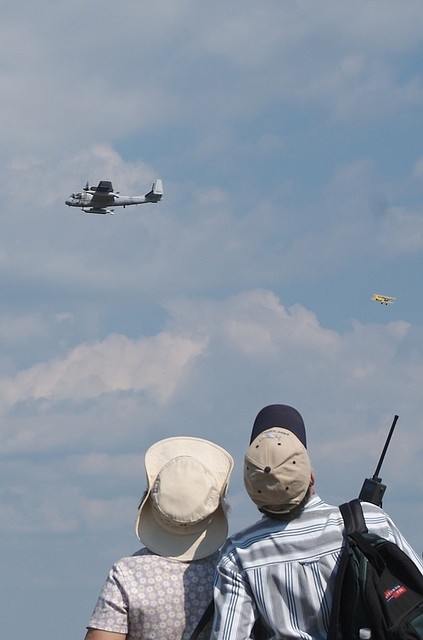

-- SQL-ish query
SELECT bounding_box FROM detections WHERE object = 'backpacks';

[326,498,422,640]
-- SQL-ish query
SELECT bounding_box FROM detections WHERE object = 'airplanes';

[65,178,162,214]
[372,294,396,305]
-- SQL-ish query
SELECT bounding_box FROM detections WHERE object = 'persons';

[83,436,233,640]
[196,404,420,640]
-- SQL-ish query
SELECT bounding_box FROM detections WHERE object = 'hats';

[135,436,234,561]
[244,404,311,515]
